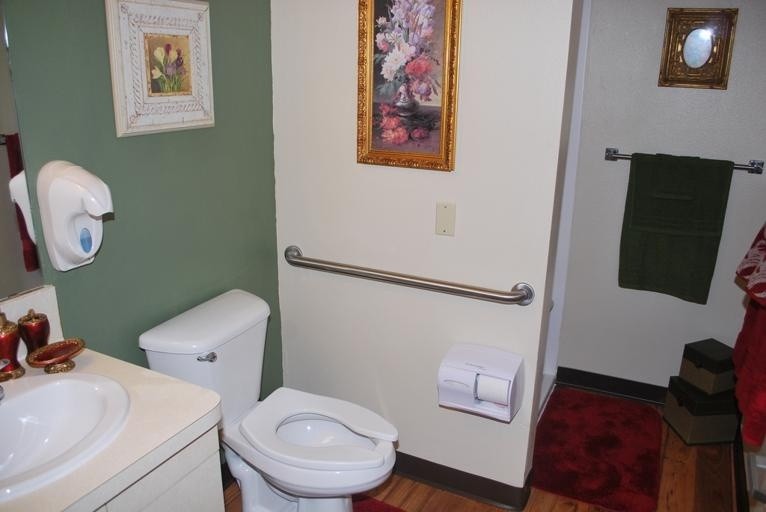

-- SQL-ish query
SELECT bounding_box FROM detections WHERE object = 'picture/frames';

[354,0,463,172]
[104,0,215,140]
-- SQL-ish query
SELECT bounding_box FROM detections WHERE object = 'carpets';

[529,384,669,512]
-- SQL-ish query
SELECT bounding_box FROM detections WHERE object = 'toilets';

[139,289,399,512]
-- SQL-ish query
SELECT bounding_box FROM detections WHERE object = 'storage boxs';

[664,328,741,448]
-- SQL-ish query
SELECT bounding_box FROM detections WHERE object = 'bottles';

[0,309,50,383]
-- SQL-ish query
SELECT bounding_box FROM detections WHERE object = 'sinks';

[0,371,129,501]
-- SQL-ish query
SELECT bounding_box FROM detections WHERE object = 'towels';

[730,217,765,448]
[616,147,736,305]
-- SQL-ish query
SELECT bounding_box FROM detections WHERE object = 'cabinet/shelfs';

[87,422,226,512]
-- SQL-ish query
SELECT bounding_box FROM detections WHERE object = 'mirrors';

[0,0,44,307]
[657,7,740,90]
